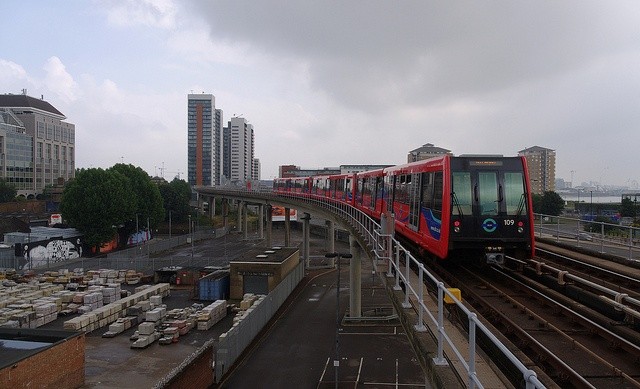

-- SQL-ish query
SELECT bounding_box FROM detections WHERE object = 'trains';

[273,154,535,261]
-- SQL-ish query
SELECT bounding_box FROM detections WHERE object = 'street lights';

[324,251,351,388]
[188,214,192,233]
[634,194,637,223]
[155,228,158,241]
[590,189,593,221]
